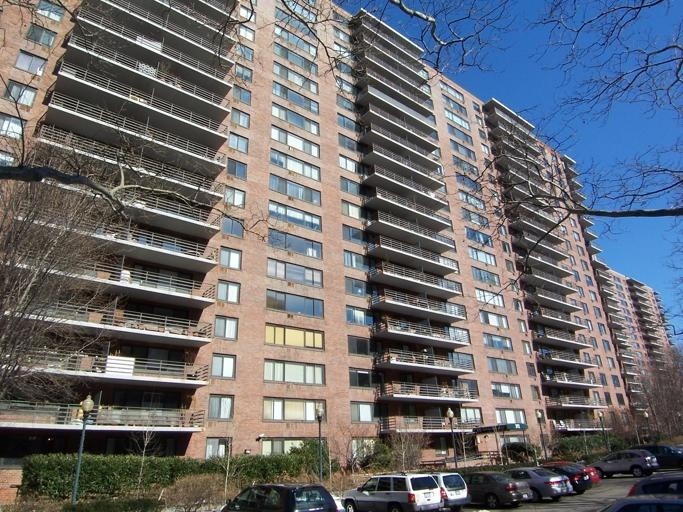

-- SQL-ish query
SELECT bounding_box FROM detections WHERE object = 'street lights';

[643,412,654,437]
[71,393,94,505]
[316,403,325,482]
[535,408,549,460]
[597,411,609,449]
[447,408,458,470]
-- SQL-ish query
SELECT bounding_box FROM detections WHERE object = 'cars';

[219,484,338,512]
